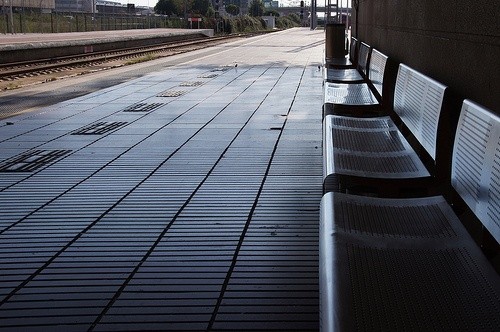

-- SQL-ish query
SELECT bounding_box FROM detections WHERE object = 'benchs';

[320,99,500,332]
[322,49,389,116]
[324,42,371,83]
[326,36,358,68]
[322,64,449,195]
